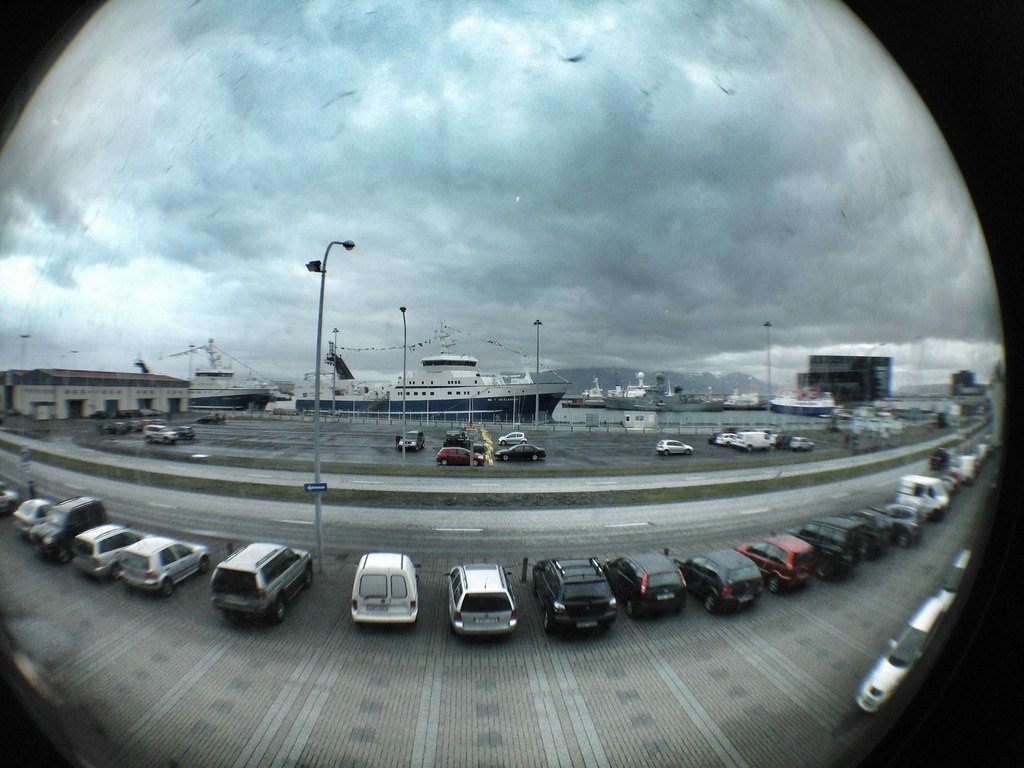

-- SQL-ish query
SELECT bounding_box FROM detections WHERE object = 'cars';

[0,488,18,515]
[791,435,816,451]
[397,430,546,465]
[707,429,779,452]
[857,547,1005,713]
[656,438,695,455]
[799,432,1000,578]
[10,498,52,536]
[98,416,198,444]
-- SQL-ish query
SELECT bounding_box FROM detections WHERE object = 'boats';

[173,336,281,415]
[288,321,574,425]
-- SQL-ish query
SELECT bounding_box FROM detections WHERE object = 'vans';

[28,495,107,564]
[348,552,420,629]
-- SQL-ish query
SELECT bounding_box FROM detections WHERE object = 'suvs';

[604,552,689,618]
[736,535,812,597]
[675,547,765,615]
[442,563,518,638]
[116,534,211,598]
[208,541,313,624]
[531,556,619,635]
[69,524,151,584]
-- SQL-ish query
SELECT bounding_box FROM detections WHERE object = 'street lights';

[400,306,409,466]
[533,318,545,431]
[328,326,342,425]
[761,319,772,430]
[304,237,357,576]
